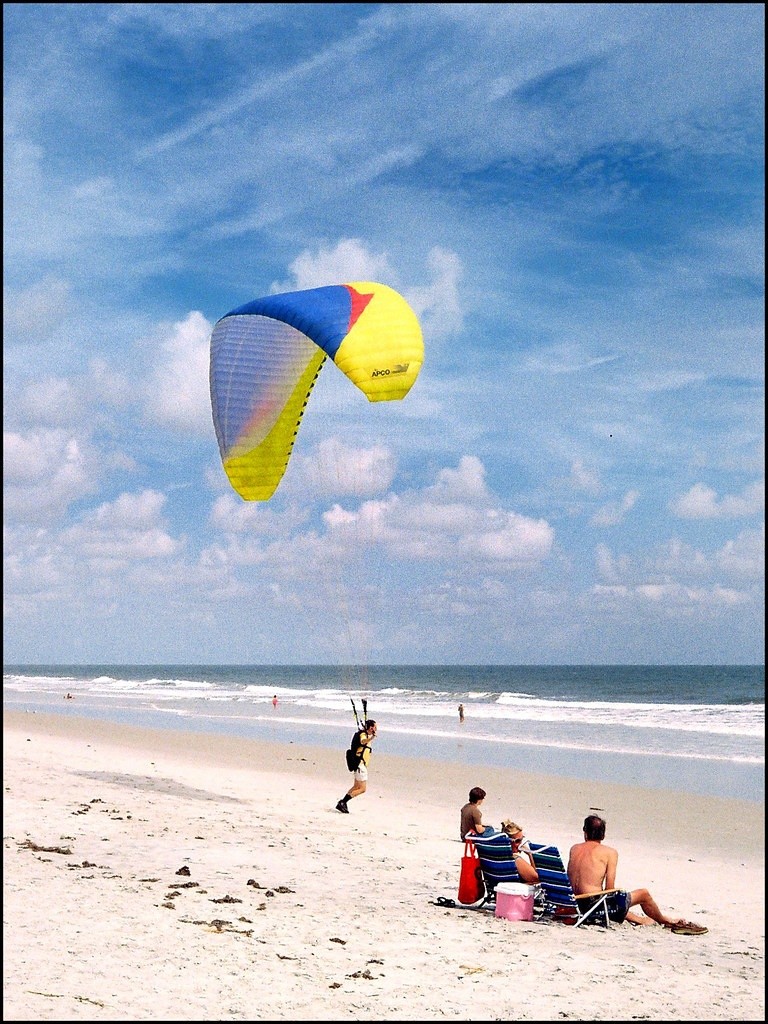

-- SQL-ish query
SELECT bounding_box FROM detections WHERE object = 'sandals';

[433,897,456,908]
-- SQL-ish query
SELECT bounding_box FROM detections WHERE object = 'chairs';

[465,830,554,921]
[518,839,619,928]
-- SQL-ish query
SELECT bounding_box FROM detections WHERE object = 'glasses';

[583,827,587,831]
[511,835,525,843]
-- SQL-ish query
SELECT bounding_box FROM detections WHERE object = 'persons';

[461,787,486,841]
[458,704,464,723]
[336,720,377,813]
[567,813,685,926]
[273,695,278,709]
[502,822,539,882]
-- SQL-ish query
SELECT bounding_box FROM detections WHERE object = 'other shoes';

[672,919,708,935]
[336,800,349,813]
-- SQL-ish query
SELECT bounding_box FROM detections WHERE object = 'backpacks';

[346,730,368,772]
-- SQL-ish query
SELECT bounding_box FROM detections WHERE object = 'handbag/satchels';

[458,839,485,904]
